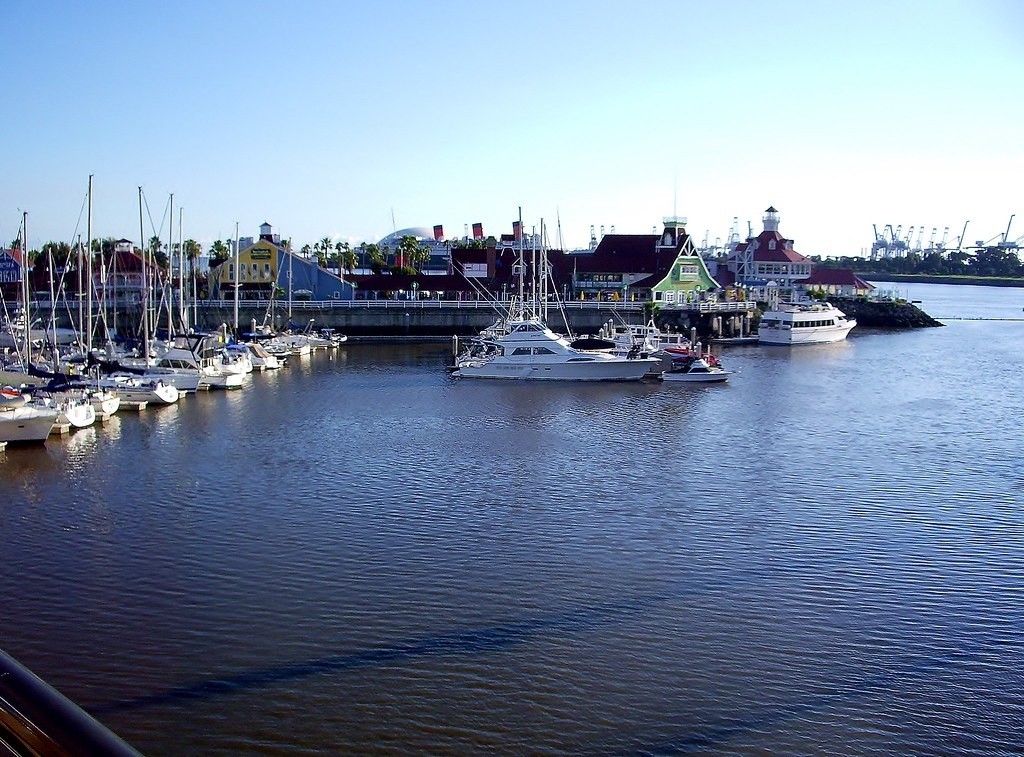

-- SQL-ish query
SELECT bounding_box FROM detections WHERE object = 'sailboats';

[451,206,692,381]
[0,174,347,447]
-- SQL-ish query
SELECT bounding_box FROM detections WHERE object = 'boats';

[661,358,732,383]
[452,320,661,381]
[757,299,857,345]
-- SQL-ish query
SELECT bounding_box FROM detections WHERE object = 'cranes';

[871,215,1024,255]
